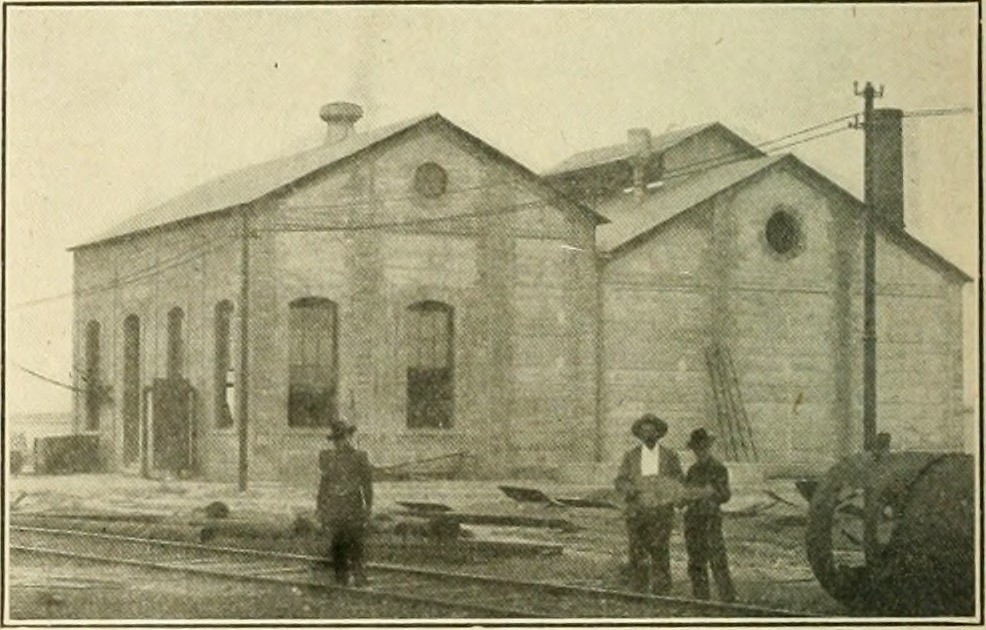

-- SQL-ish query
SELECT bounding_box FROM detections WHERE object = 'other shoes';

[360,576,375,586]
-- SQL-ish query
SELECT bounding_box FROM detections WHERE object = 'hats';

[328,422,356,441]
[687,427,716,449]
[632,414,667,438]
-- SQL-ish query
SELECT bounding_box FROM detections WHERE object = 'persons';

[683,428,736,602]
[317,421,374,591]
[614,413,684,597]
